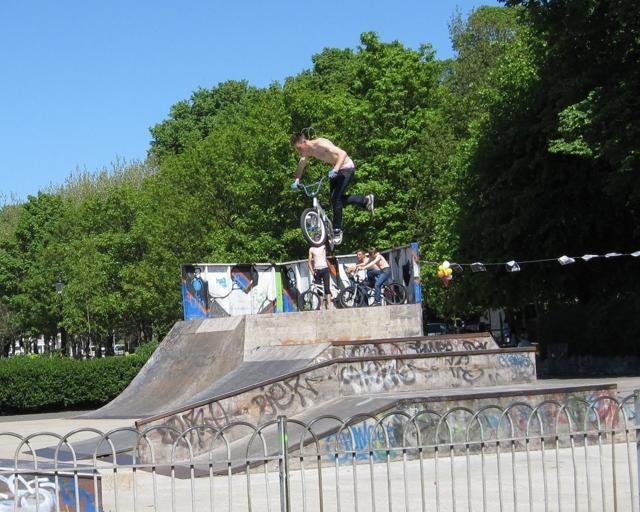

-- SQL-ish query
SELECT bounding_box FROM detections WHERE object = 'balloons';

[437,260,455,283]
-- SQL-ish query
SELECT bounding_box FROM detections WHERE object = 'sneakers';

[333,229,343,245]
[373,302,381,305]
[364,194,375,218]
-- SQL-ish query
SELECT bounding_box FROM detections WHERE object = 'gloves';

[291,182,301,189]
[329,171,337,178]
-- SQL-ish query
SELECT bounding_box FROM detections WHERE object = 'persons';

[307,234,333,310]
[517,333,531,346]
[289,132,374,245]
[357,246,392,306]
[345,248,380,307]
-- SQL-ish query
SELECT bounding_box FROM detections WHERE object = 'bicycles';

[289,174,343,253]
[297,266,407,311]
